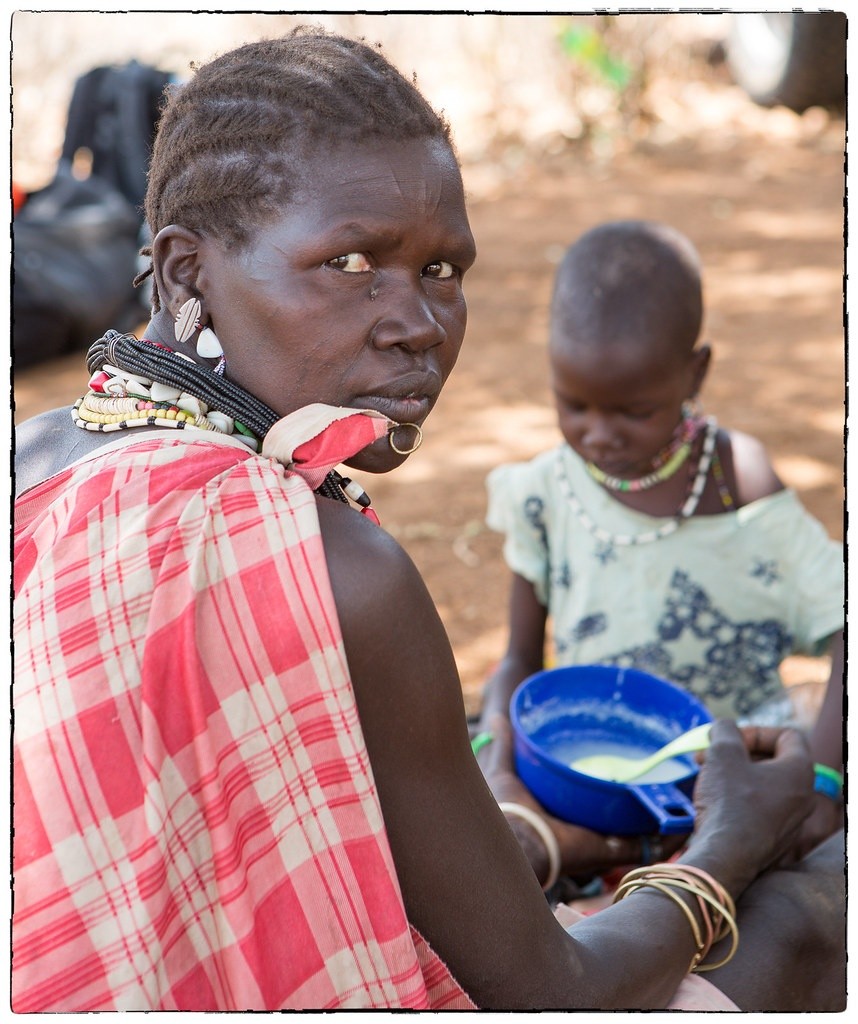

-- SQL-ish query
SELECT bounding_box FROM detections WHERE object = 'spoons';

[571,721,713,782]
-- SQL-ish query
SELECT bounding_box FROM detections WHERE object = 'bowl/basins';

[508,663,715,838]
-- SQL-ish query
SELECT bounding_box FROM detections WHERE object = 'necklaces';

[552,411,722,546]
[73,329,380,522]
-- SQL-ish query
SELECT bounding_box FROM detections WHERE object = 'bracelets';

[815,763,843,804]
[619,866,740,975]
[497,795,559,893]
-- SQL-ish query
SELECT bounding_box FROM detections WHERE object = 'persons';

[8,23,850,1014]
[10,176,143,422]
[456,219,846,900]
